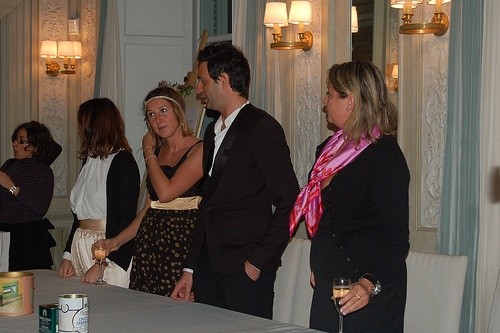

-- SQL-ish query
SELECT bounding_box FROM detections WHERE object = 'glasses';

[15,138,31,144]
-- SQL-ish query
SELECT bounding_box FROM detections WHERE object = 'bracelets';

[143,147,153,154]
[145,155,157,161]
[9,186,17,194]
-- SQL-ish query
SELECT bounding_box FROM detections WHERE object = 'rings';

[356,294,361,299]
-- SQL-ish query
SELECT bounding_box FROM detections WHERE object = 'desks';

[0,269,325,333]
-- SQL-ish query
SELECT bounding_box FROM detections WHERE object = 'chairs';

[403,250,467,333]
[274,239,313,328]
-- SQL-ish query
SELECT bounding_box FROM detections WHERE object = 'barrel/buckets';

[57,293,90,333]
[0,271,34,316]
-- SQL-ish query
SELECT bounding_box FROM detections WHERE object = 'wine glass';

[332,277,352,333]
[92,239,107,284]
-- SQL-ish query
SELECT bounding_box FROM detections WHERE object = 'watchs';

[362,274,381,297]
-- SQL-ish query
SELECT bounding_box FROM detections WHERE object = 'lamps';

[264,1,314,52]
[391,65,400,92]
[350,5,358,33]
[391,0,450,37]
[41,40,82,75]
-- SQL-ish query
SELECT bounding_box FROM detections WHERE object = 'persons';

[170,41,302,320]
[0,120,63,271]
[58,98,141,289]
[91,85,203,295]
[289,60,410,333]
